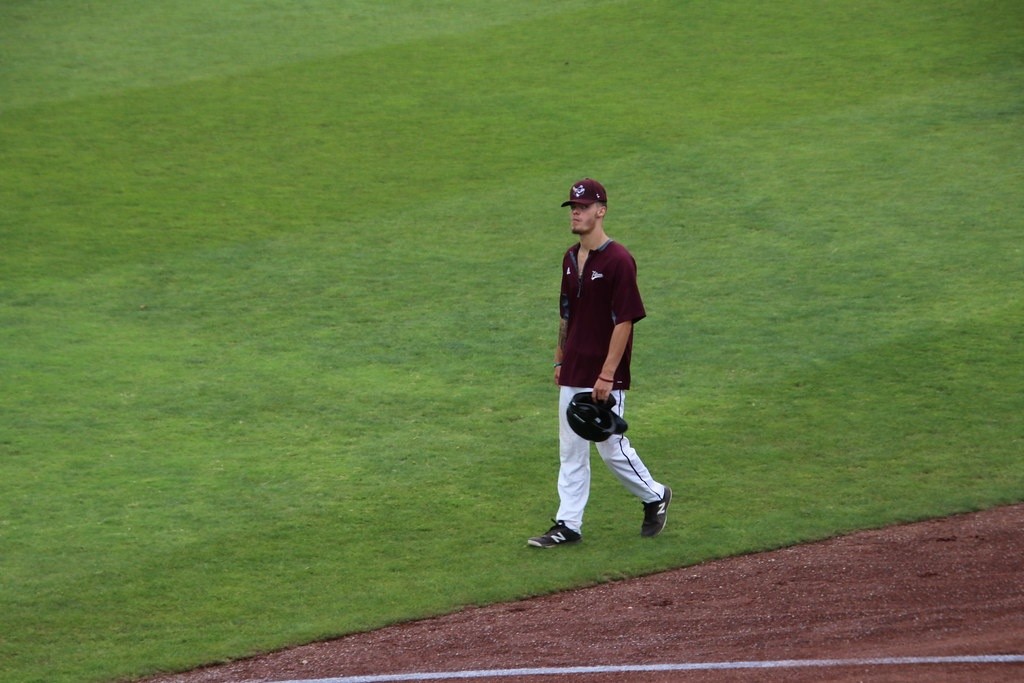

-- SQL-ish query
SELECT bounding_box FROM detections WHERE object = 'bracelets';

[598,375,614,383]
[553,361,562,368]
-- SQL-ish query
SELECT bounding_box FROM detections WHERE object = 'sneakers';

[528,518,582,548]
[640,486,672,538]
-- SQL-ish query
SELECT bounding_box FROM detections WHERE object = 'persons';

[525,177,671,547]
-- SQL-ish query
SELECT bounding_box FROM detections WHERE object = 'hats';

[561,177,607,207]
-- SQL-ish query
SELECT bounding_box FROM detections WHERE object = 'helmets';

[567,391,628,442]
